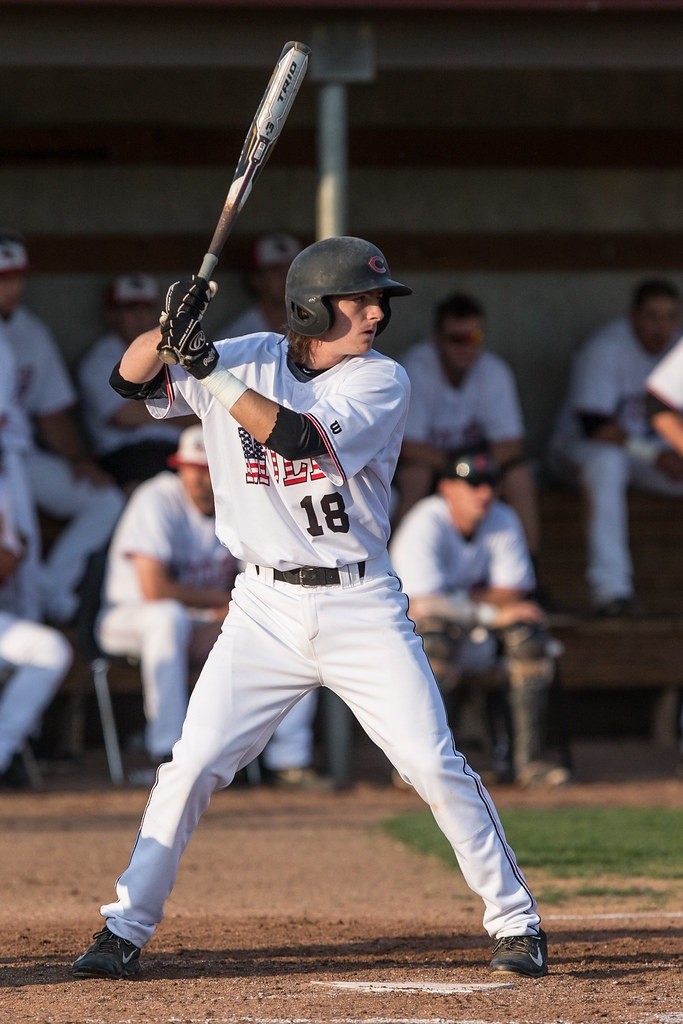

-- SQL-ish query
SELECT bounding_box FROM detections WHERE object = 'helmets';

[285,236,413,337]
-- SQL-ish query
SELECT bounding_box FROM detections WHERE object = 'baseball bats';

[158,40,315,368]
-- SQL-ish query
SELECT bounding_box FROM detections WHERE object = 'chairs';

[72,530,267,792]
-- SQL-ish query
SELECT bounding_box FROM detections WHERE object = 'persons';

[0,235,324,791]
[389,287,543,564]
[388,444,564,789]
[550,277,683,621]
[71,235,549,976]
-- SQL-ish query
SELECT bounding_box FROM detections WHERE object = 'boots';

[509,678,572,790]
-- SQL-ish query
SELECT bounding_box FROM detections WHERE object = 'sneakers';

[487,926,548,979]
[71,925,140,980]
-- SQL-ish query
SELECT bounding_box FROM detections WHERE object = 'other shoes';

[599,600,645,618]
[270,766,343,795]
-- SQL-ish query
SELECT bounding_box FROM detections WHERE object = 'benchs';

[0,445,683,754]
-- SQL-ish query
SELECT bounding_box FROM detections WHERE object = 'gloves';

[156,311,219,380]
[165,274,218,323]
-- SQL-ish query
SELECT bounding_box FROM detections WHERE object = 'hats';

[444,452,504,487]
[167,424,208,468]
[249,233,301,268]
[108,271,160,306]
[0,233,28,275]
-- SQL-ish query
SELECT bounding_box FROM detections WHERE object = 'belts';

[255,560,366,589]
